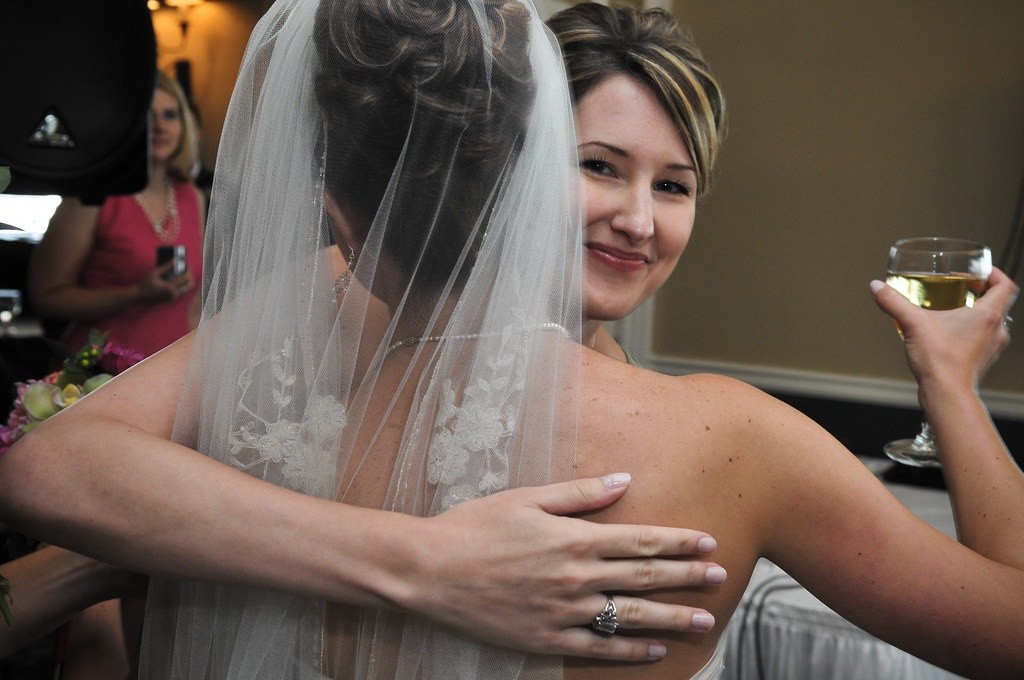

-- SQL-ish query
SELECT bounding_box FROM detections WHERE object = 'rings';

[593,594,617,633]
[178,285,187,294]
[1003,315,1014,327]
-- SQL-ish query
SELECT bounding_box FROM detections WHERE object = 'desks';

[724,454,966,679]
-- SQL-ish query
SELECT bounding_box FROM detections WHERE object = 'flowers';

[0,327,146,454]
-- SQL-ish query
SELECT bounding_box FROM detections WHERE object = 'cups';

[156,244,186,282]
[0,289,21,325]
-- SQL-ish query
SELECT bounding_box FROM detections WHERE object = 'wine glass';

[883,237,992,468]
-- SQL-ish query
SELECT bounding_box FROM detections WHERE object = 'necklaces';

[384,323,570,353]
[134,180,181,242]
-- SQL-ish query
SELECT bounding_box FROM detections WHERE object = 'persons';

[126,1,1024,679]
[0,71,215,680]
[0,1,738,679]
